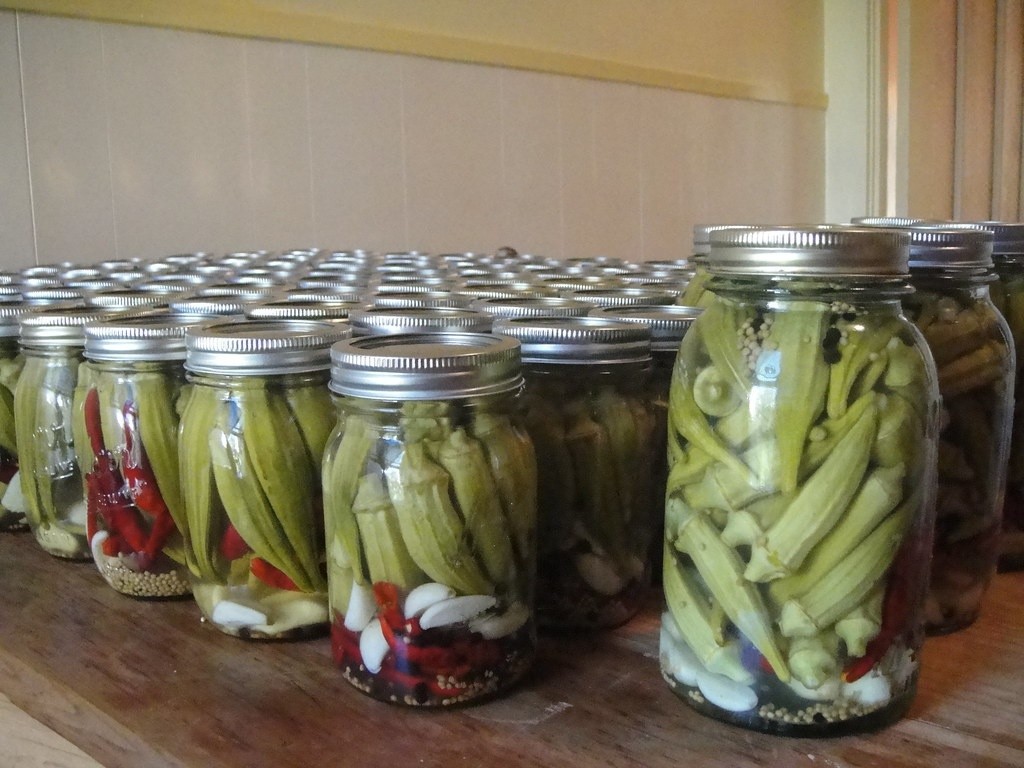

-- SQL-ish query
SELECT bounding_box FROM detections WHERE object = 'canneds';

[1,219,1024,733]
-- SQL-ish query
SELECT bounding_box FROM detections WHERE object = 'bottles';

[0,248,707,708]
[659,216,1024,740]
